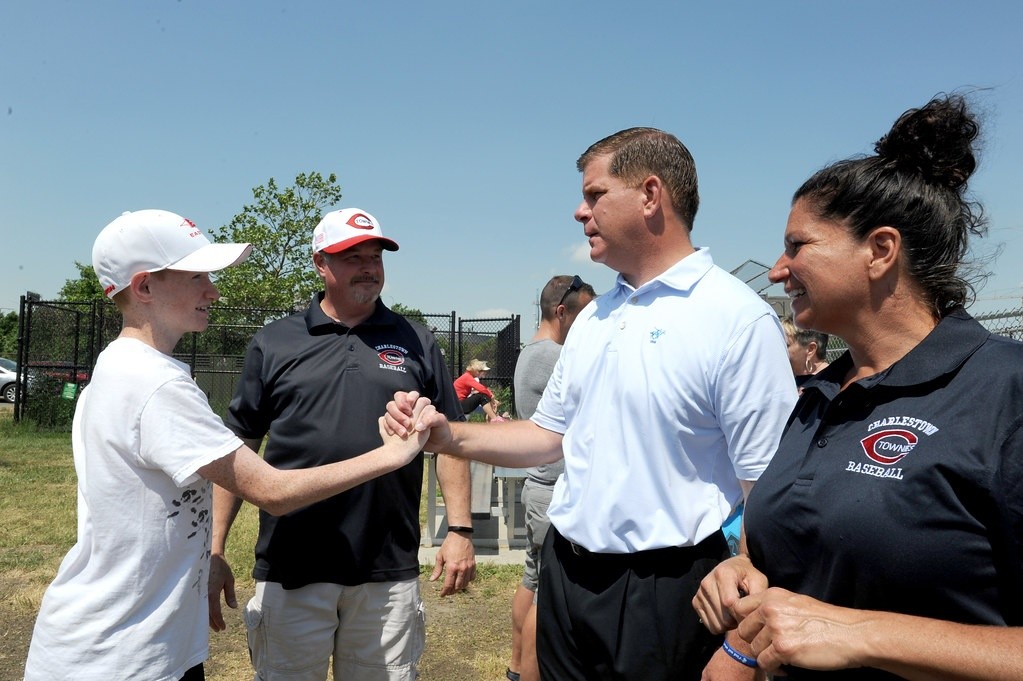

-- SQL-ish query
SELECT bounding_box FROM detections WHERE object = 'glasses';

[556,275,584,314]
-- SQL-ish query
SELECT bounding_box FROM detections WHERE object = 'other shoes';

[490,415,507,423]
[506,668,520,681]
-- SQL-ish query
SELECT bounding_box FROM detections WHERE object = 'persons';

[383,128,799,681]
[505,275,594,681]
[688,96,1023,681]
[719,311,831,557]
[21,208,437,681]
[452,358,507,423]
[207,208,475,681]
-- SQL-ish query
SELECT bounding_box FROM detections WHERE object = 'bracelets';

[447,525,474,534]
[722,641,757,668]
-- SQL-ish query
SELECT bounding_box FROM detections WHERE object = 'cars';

[0,357,38,403]
[47,367,90,384]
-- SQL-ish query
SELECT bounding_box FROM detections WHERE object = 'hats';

[91,210,252,299]
[466,359,490,371]
[312,208,399,254]
